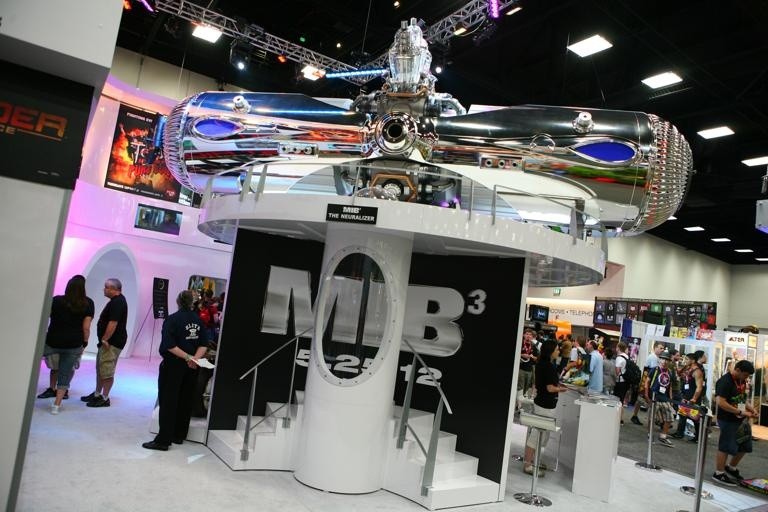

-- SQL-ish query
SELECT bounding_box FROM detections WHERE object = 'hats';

[659,352,673,361]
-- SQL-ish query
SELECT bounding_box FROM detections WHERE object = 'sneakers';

[724,466,744,480]
[712,472,737,486]
[50,402,62,415]
[86,395,110,407]
[620,415,698,448]
[63,390,68,399]
[37,387,57,399]
[81,392,101,402]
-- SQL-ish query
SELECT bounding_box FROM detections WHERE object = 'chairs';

[514,411,562,507]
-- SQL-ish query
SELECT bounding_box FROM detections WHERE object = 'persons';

[142,290,209,451]
[193,287,226,417]
[43,277,92,414]
[38,274,95,399]
[80,278,128,407]
[515,328,760,487]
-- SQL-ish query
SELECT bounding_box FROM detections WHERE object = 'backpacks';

[617,355,641,385]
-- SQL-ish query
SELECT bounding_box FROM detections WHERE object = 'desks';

[545,384,622,504]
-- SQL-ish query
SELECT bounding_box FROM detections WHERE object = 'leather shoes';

[143,441,168,451]
